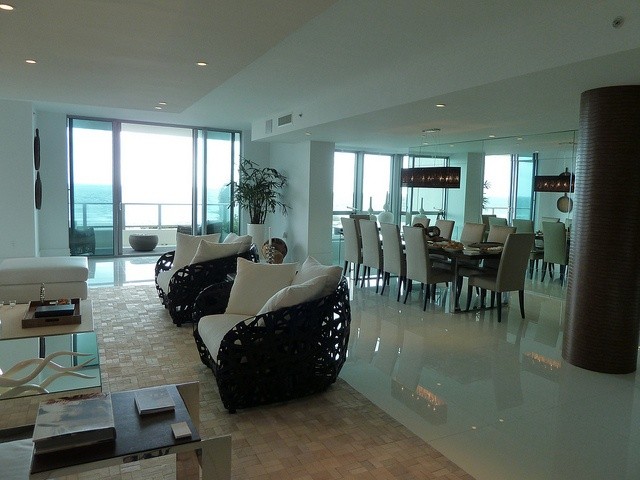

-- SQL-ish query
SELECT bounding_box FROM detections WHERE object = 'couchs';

[193,267,350,414]
[69,226,96,257]
[177,219,229,243]
[155,243,258,327]
[0,255,89,305]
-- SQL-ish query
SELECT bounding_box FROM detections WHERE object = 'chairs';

[352,309,380,365]
[489,217,508,228]
[513,219,554,280]
[540,221,570,287]
[358,218,389,293]
[376,312,402,373]
[458,222,486,296]
[350,214,370,279]
[465,232,536,323]
[389,315,430,390]
[339,217,363,286]
[565,217,571,276]
[380,221,412,303]
[433,218,455,288]
[481,214,496,242]
[475,224,518,297]
[401,224,452,312]
[412,217,431,228]
[538,216,561,279]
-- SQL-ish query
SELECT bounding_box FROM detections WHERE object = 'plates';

[442,246,462,252]
[430,248,443,251]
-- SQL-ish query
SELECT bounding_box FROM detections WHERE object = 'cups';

[9,299,16,309]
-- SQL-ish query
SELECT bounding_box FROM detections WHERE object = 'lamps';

[534,174,574,192]
[400,166,462,188]
[522,350,564,378]
[389,377,447,426]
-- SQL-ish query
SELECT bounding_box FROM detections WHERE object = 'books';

[134,386,175,416]
[33,304,75,317]
[32,392,116,456]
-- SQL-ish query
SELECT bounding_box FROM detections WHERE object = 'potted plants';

[225,153,294,249]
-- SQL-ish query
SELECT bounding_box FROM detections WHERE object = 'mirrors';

[404,129,577,299]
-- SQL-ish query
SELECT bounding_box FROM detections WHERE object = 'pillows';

[292,256,343,305]
[253,274,328,335]
[222,231,252,254]
[189,239,242,272]
[224,256,299,315]
[172,230,222,270]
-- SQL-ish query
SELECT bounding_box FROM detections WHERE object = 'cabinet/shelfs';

[1,381,233,480]
